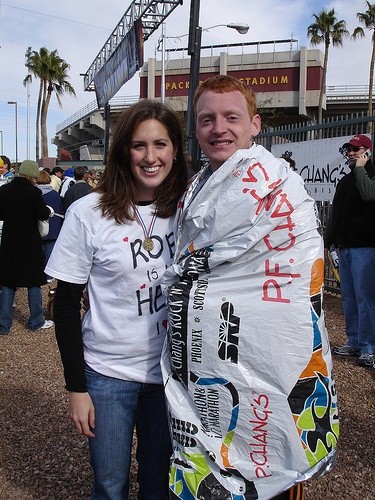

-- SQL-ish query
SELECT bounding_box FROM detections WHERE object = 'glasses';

[347,147,366,153]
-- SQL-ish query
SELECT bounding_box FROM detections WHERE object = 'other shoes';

[38,320,54,330]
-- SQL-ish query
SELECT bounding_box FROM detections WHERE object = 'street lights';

[8,101,17,173]
[161,23,250,105]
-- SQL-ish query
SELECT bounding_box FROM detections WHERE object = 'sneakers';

[357,352,375,366]
[333,341,362,356]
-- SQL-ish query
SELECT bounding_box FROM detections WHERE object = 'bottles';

[330,248,339,268]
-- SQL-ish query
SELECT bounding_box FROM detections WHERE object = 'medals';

[143,239,154,252]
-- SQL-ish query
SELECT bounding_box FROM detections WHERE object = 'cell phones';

[363,149,371,158]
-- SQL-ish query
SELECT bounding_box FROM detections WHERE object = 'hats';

[342,134,372,151]
[65,168,74,176]
[19,160,40,179]
[51,167,63,174]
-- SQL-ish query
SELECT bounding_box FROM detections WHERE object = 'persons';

[43,98,188,500]
[1,156,102,284]
[161,75,340,500]
[0,160,53,336]
[325,135,375,368]
